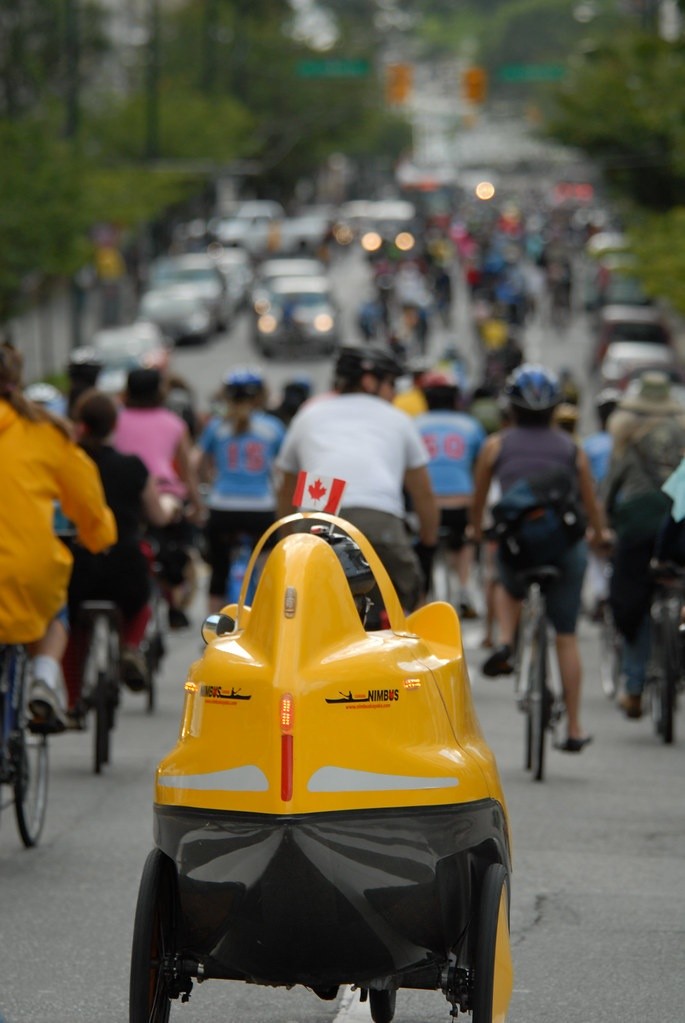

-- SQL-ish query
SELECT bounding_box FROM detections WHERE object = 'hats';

[617,373,682,416]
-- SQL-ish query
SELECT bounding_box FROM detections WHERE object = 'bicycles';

[462,522,612,786]
[60,505,685,773]
[0,531,91,849]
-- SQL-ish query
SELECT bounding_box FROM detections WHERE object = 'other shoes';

[564,733,594,748]
[28,679,68,731]
[166,605,190,630]
[119,649,149,693]
[616,695,643,717]
[482,643,514,676]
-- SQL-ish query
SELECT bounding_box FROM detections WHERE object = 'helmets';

[67,346,102,366]
[414,371,455,388]
[337,328,407,374]
[23,381,61,404]
[503,364,562,411]
[225,371,263,387]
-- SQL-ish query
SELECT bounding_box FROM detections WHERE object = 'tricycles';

[127,511,514,1023]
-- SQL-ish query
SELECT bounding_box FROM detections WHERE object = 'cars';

[74,320,178,394]
[135,195,408,362]
[574,203,685,428]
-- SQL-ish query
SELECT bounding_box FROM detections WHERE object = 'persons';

[269,344,441,613]
[464,363,616,754]
[17,201,685,734]
[0,340,114,735]
